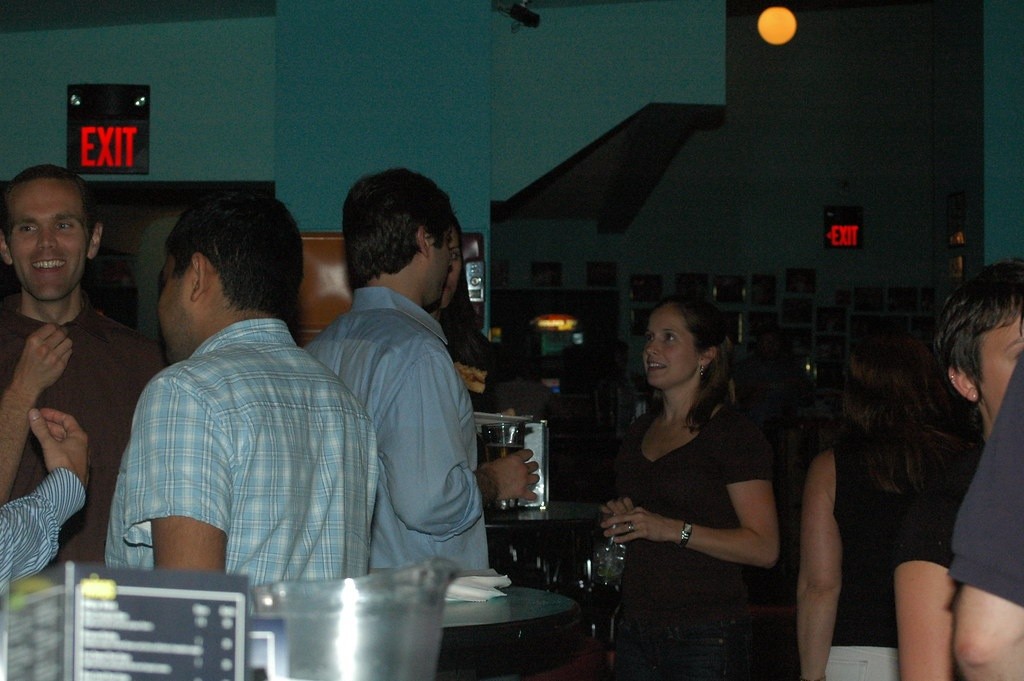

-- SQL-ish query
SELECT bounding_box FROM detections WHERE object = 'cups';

[591,513,627,585]
[482,422,525,511]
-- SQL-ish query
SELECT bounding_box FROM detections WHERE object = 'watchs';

[679,521,692,547]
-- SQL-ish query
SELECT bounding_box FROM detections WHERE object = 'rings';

[628,522,634,533]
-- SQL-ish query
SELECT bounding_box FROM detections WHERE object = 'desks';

[485,502,606,594]
[439,586,583,677]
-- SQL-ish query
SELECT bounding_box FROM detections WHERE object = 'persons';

[431,208,515,421]
[1,162,166,565]
[1,408,93,602]
[598,293,781,681]
[795,334,982,680]
[102,186,380,579]
[739,328,812,410]
[944,352,1024,681]
[301,167,541,579]
[891,255,1024,680]
